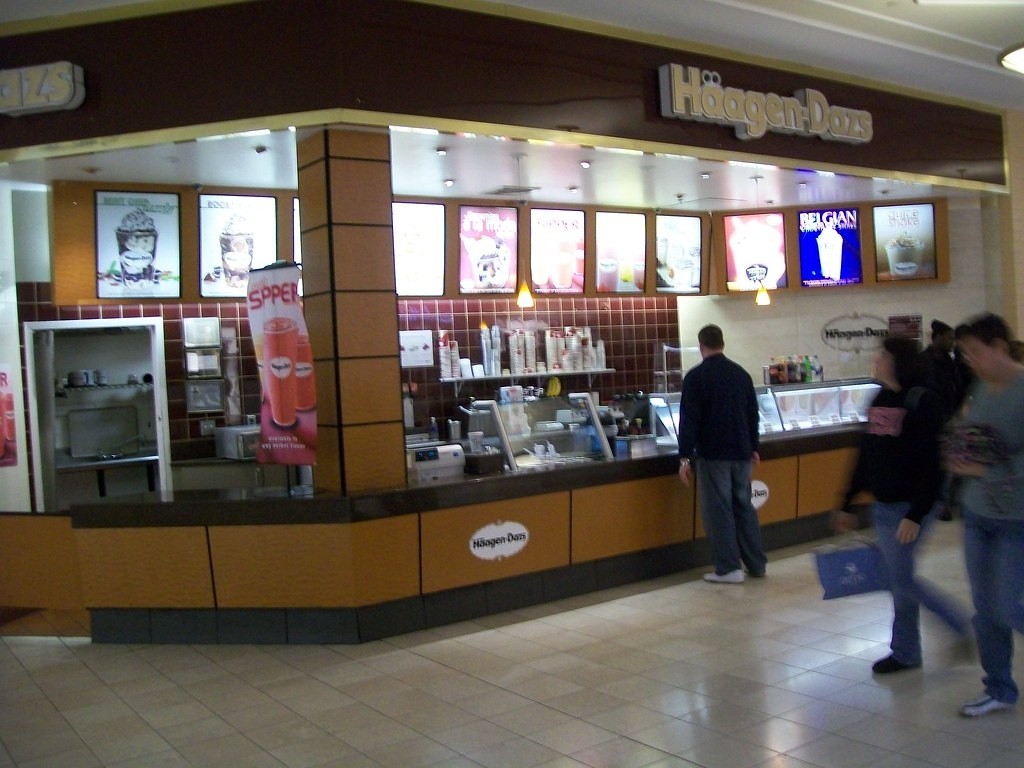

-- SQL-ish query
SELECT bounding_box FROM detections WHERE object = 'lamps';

[750,174,771,307]
[510,152,535,308]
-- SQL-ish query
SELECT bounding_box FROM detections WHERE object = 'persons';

[835,333,978,674]
[917,313,1024,716]
[678,324,768,583]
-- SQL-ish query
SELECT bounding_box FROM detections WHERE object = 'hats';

[930,320,951,341]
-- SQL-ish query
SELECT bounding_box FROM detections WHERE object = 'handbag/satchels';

[814,527,892,601]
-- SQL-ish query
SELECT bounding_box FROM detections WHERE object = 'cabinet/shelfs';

[468,393,614,468]
[23,317,174,506]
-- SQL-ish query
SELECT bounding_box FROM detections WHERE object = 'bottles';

[769,355,823,384]
[403,395,414,428]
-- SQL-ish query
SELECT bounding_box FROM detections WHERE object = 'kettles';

[470,255,508,288]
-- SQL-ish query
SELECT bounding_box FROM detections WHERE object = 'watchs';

[680,458,691,465]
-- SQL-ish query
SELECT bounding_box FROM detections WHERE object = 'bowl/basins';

[567,424,581,431]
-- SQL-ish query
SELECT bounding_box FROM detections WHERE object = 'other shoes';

[957,693,1015,716]
[703,569,746,584]
[746,565,767,579]
[873,654,921,674]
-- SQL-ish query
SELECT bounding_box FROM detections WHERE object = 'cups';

[598,259,618,292]
[673,270,692,290]
[467,432,485,453]
[129,373,138,384]
[293,335,317,412]
[438,325,607,379]
[114,227,158,291]
[884,243,925,275]
[815,237,843,278]
[67,369,108,386]
[449,420,462,439]
[262,319,298,426]
[534,445,555,455]
[728,239,782,289]
[218,232,253,288]
